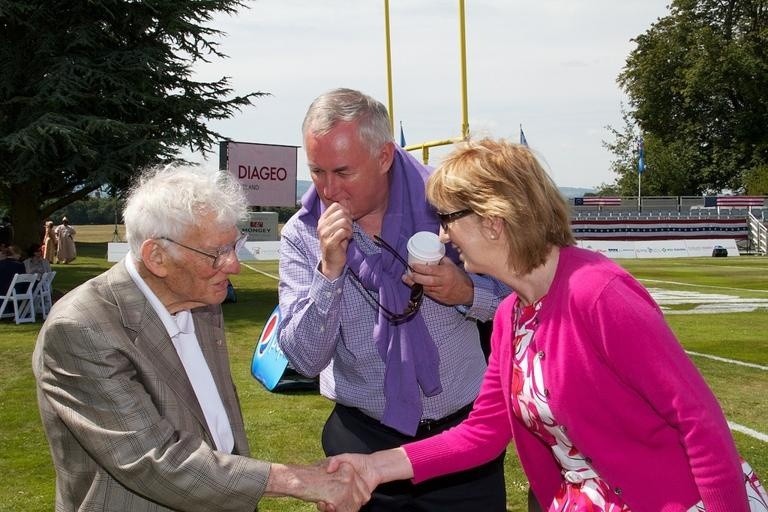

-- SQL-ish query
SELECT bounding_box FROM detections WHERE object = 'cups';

[407,231,446,278]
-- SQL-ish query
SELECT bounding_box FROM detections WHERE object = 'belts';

[346,403,474,440]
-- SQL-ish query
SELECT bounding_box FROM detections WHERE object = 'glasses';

[348,234,424,322]
[155,232,249,269]
[434,207,473,230]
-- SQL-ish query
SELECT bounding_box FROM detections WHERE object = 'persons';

[55,216,75,264]
[0,244,28,322]
[0,237,29,262]
[30,161,370,511]
[315,134,751,512]
[43,221,58,263]
[274,83,515,512]
[23,244,52,294]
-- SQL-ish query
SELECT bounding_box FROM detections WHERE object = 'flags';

[400,124,406,148]
[638,138,645,176]
[519,127,528,145]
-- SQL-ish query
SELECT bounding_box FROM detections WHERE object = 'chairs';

[0,270,56,325]
